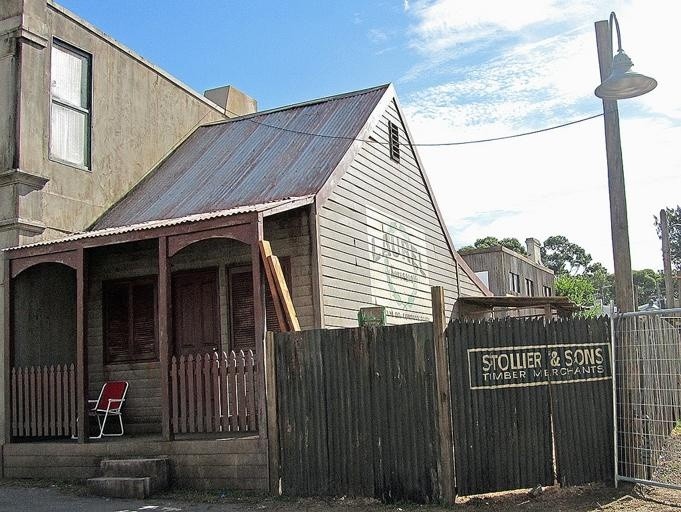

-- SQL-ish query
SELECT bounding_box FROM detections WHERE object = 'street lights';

[593,11,660,315]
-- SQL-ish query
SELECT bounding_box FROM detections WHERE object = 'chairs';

[71,380,129,440]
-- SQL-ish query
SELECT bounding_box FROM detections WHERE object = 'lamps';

[594,11,658,101]
